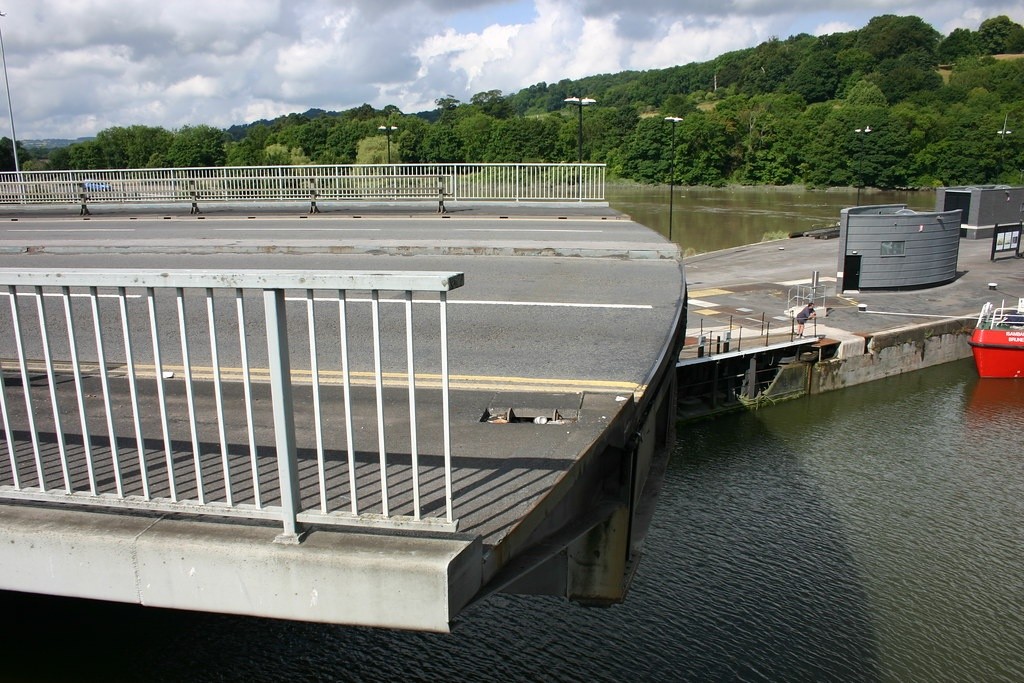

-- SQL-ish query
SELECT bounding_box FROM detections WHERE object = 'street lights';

[563,97,597,163]
[664,116,683,241]
[379,125,398,164]
[995,131,1012,183]
[855,128,872,207]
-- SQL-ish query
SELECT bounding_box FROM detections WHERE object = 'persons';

[795,303,816,338]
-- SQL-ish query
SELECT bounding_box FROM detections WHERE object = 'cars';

[83,181,111,192]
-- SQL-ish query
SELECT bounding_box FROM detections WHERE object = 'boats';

[967,299,1024,379]
